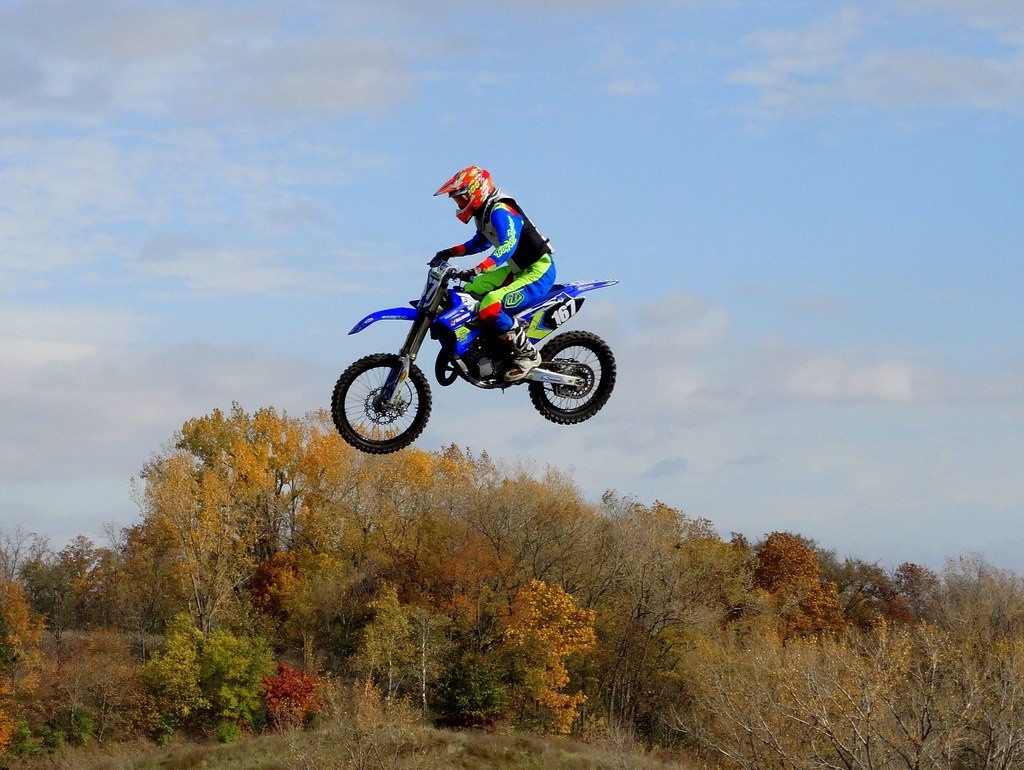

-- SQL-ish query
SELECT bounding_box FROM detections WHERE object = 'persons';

[429,166,556,382]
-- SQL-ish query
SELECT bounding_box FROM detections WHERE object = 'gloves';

[432,249,453,264]
[458,268,478,282]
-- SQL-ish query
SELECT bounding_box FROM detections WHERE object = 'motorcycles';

[329,263,621,455]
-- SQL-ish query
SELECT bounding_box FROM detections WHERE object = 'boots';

[498,317,543,383]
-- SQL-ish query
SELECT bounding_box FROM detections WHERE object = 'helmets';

[433,164,495,225]
